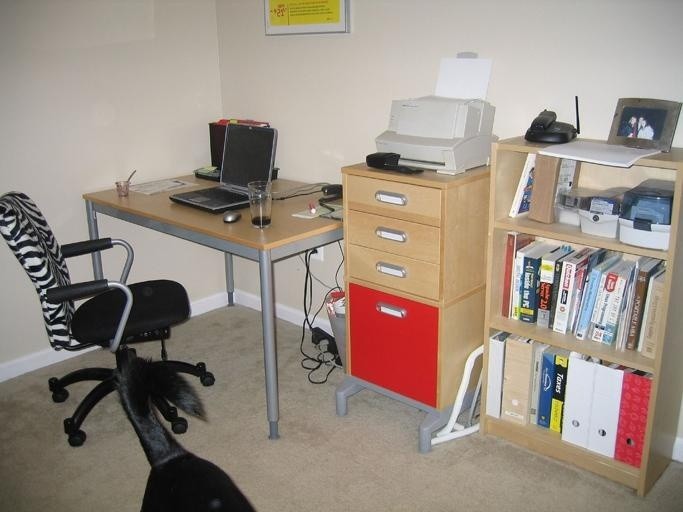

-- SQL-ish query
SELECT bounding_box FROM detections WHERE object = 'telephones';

[525,96,580,143]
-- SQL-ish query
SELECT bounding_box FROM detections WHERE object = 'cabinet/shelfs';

[480,135,683,498]
[335,162,490,454]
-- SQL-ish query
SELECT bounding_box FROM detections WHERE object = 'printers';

[374,95,499,175]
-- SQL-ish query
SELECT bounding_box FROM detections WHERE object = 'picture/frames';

[607,98,683,152]
[264,0,350,36]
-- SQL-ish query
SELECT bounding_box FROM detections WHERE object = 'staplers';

[318,184,342,203]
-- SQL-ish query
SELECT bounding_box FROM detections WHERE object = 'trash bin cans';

[326,291,346,373]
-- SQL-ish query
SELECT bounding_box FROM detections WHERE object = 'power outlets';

[308,246,324,262]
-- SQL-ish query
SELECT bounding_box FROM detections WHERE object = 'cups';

[114,181,131,199]
[246,180,272,229]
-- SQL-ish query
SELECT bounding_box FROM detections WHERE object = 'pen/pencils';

[126,171,136,183]
[309,202,315,214]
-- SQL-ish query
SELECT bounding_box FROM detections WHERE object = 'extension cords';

[310,327,338,355]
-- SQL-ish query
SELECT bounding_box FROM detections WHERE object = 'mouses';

[224,210,242,222]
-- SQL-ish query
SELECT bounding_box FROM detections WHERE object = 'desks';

[83,168,343,439]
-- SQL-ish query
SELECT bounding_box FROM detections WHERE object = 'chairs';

[0,191,215,447]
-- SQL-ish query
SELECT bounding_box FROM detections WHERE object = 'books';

[502,231,666,360]
[487,331,654,468]
[291,199,343,221]
[538,139,661,169]
[507,152,534,221]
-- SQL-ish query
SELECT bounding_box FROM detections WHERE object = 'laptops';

[168,124,278,213]
